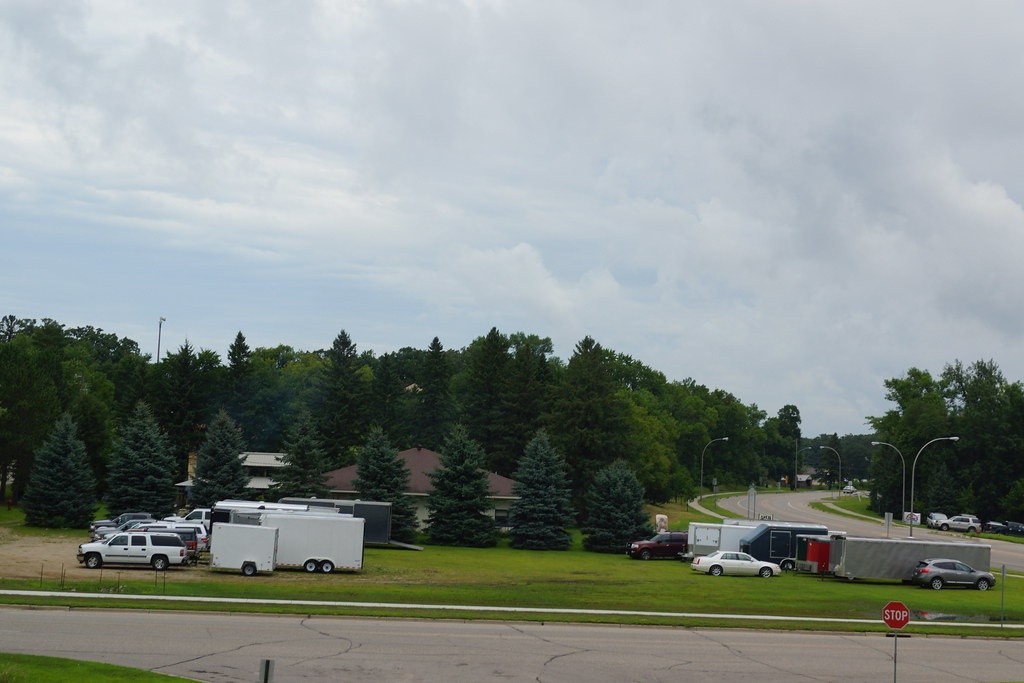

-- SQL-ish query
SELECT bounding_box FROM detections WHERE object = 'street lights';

[910,437,960,537]
[156,316,166,363]
[820,445,842,499]
[700,436,730,500]
[795,438,812,489]
[871,441,905,523]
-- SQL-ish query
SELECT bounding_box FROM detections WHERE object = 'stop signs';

[881,601,910,629]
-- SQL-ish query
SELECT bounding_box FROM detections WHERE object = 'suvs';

[77,531,188,571]
[940,514,982,533]
[626,530,688,561]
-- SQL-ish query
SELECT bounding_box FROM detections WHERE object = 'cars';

[984,521,1007,534]
[1005,521,1024,535]
[92,509,212,559]
[690,551,782,578]
[843,486,857,494]
[911,558,997,591]
[926,512,949,529]
